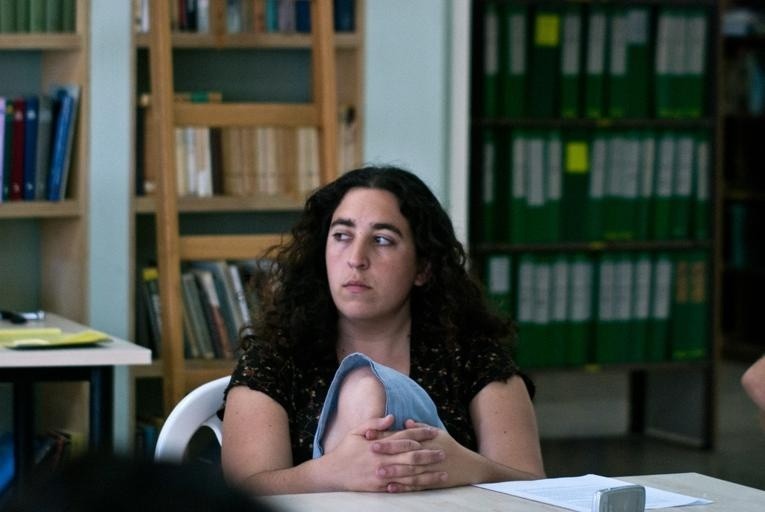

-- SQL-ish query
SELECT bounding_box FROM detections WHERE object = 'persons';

[219,162,551,497]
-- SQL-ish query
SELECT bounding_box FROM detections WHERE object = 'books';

[482,130,711,244]
[27,426,87,474]
[139,93,359,198]
[134,0,357,36]
[479,2,708,120]
[482,252,708,364]
[136,261,254,361]
[0,2,82,203]
